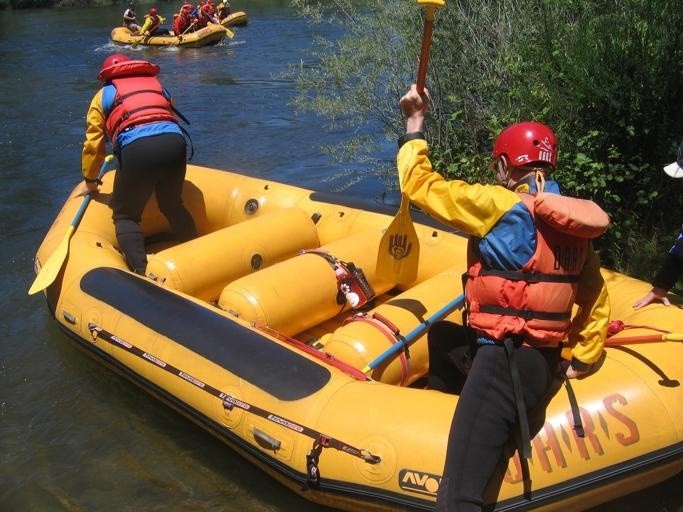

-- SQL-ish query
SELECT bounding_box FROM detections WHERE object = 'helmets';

[182,0,227,15]
[102,54,128,69]
[493,121,557,172]
[149,7,157,14]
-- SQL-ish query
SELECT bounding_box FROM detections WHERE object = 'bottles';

[346,262,376,303]
[334,267,369,310]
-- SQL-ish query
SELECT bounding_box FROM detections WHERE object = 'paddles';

[376,1,447,282]
[28,155,114,294]
[131,19,165,48]
[202,9,234,39]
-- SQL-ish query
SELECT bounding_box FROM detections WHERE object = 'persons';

[629,145,682,314]
[120,1,142,36]
[392,81,610,512]
[171,0,230,39]
[138,6,171,36]
[75,55,196,280]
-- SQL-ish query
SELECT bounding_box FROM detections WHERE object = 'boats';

[109,10,248,48]
[35,161,681,511]
